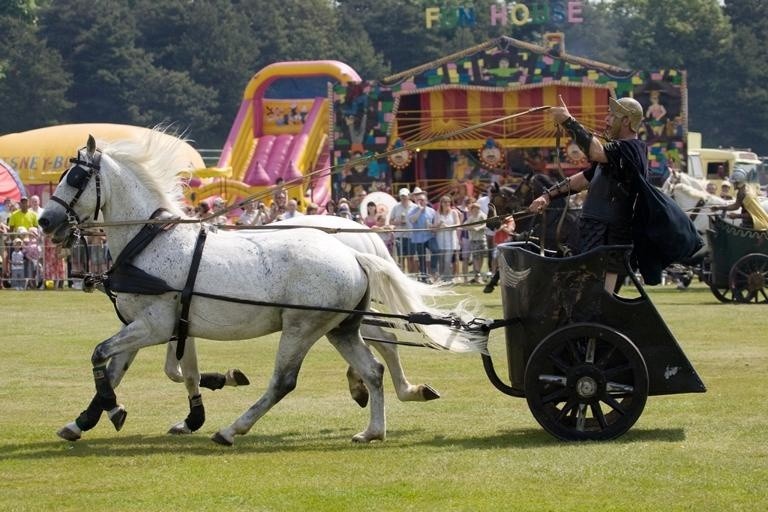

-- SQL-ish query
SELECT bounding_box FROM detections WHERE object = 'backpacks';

[628,172,702,285]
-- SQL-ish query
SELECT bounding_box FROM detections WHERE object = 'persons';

[526,92,650,249]
[0,195,113,291]
[306,182,516,285]
[708,167,768,231]
[185,178,307,227]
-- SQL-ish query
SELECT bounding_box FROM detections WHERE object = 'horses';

[38,117,493,447]
[661,165,768,290]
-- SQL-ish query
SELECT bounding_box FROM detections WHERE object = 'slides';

[213,101,331,224]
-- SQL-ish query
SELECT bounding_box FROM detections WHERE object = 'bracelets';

[559,117,594,161]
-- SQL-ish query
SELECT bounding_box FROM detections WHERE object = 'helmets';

[605,96,644,131]
